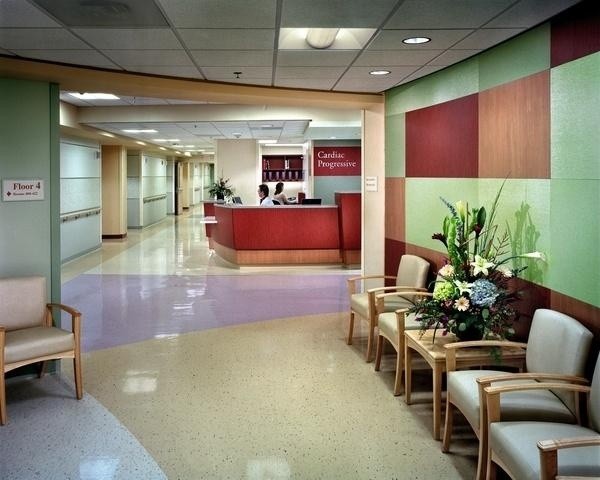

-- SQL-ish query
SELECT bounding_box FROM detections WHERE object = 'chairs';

[1,274,83,426]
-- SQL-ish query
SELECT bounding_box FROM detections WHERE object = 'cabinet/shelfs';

[263,155,305,182]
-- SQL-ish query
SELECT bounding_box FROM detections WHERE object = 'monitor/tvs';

[232,196,242,203]
[302,199,321,205]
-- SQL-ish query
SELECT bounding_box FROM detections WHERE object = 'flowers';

[209,176,236,200]
[394,169,551,368]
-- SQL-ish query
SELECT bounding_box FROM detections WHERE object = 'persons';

[273,182,298,206]
[254,183,274,206]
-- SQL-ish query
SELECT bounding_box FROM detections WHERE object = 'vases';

[456,323,484,341]
[217,193,224,204]
